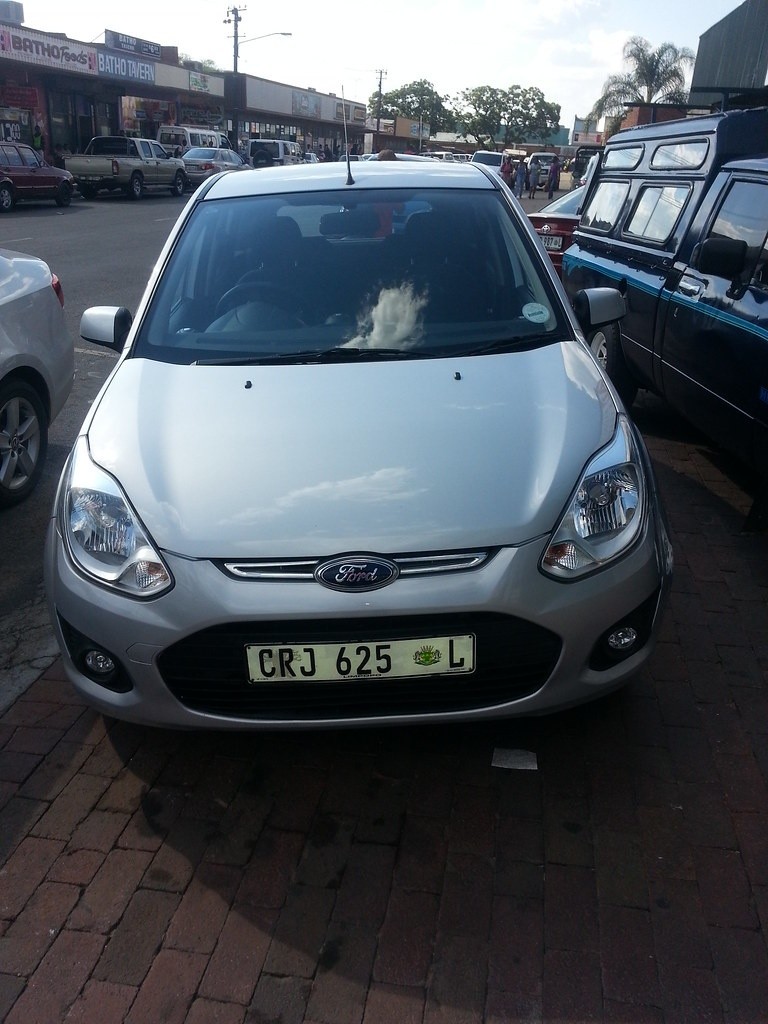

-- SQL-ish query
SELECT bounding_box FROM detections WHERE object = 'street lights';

[232,32,292,153]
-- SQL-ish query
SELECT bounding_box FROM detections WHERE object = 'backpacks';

[174,145,183,158]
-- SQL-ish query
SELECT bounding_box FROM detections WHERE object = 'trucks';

[569,145,606,191]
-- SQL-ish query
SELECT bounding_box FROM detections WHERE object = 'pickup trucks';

[563,104,768,510]
[64,134,187,200]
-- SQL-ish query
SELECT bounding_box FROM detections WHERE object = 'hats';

[253,150,273,168]
[377,149,402,161]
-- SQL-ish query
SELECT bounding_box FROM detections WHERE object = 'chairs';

[293,236,343,309]
[401,213,449,324]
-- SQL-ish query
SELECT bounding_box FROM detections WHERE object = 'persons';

[174,139,187,158]
[241,139,419,239]
[31,126,45,166]
[499,156,561,200]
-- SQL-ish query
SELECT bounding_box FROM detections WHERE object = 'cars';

[0,250,77,510]
[305,152,321,163]
[525,184,589,272]
[341,152,442,235]
[0,140,74,212]
[180,148,252,184]
[44,161,674,733]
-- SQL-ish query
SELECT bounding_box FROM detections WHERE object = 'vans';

[339,149,562,190]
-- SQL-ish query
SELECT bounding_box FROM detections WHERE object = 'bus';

[248,138,308,168]
[155,125,236,158]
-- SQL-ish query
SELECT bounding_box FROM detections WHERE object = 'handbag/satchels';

[544,183,549,192]
